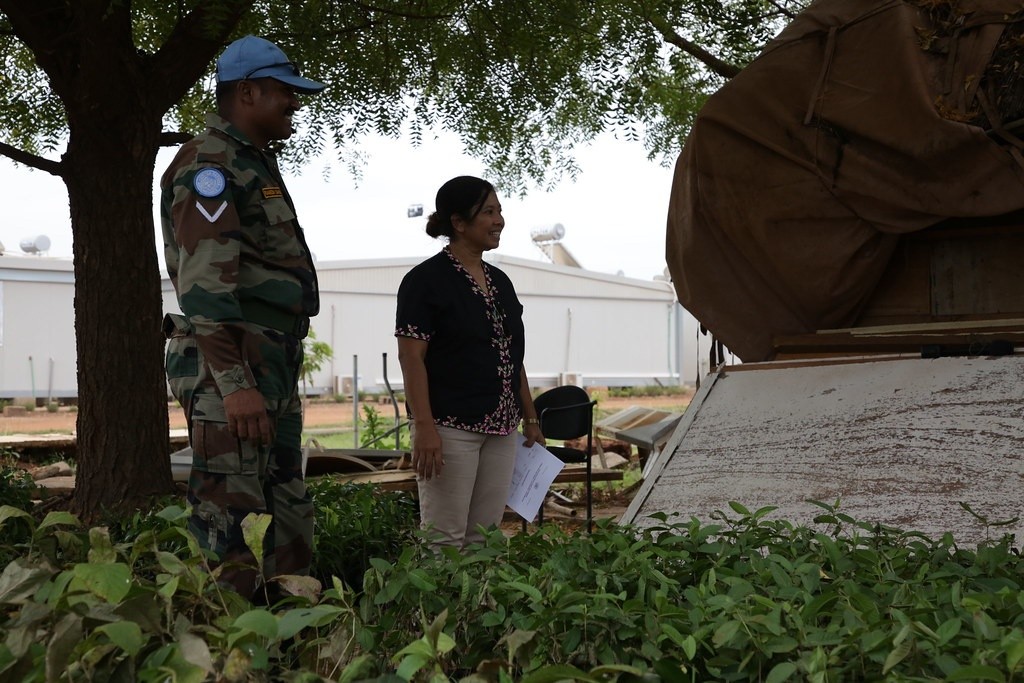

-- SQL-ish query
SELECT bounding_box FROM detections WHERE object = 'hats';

[215,35,325,95]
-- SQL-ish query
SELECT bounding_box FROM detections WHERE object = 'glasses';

[244,59,300,78]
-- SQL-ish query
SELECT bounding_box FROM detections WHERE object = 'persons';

[161,35,327,683]
[395,176,545,558]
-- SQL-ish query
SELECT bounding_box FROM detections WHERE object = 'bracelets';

[522,418,539,427]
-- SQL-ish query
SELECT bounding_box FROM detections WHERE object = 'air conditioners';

[333,375,363,398]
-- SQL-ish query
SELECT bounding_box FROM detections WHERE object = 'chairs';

[522,385,597,541]
